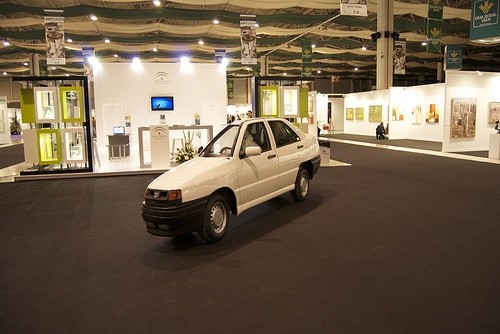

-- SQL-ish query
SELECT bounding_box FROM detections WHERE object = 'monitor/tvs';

[113,126,125,134]
[151,96,174,111]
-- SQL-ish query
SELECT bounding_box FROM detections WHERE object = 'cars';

[139,117,321,244]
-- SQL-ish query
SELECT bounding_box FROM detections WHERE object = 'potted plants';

[10,112,21,142]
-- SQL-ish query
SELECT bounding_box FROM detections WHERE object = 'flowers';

[170,130,198,164]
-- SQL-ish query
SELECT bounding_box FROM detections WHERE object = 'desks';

[138,126,213,168]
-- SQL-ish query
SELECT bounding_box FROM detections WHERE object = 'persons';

[246,110,252,118]
[376,122,385,140]
